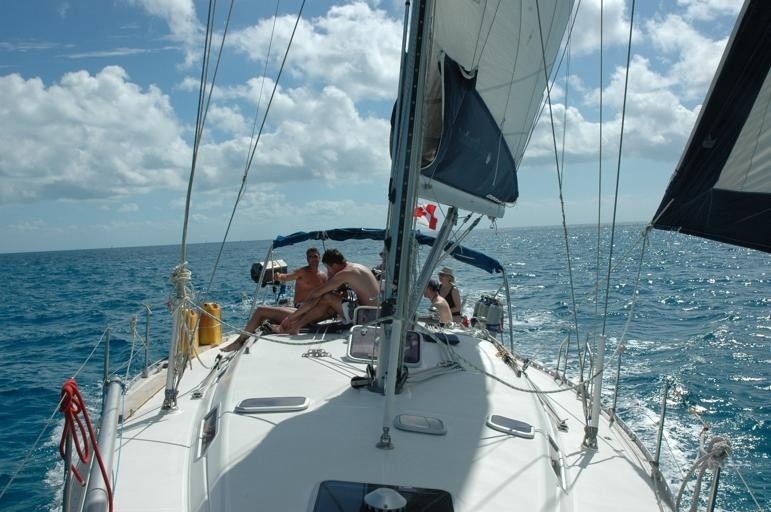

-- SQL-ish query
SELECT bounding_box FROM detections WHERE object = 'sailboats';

[0,0,771,512]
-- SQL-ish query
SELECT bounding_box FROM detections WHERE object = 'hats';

[438,266,456,283]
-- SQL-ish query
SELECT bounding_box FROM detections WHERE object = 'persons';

[219,248,463,351]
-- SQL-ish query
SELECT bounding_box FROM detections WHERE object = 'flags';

[412,200,440,232]
